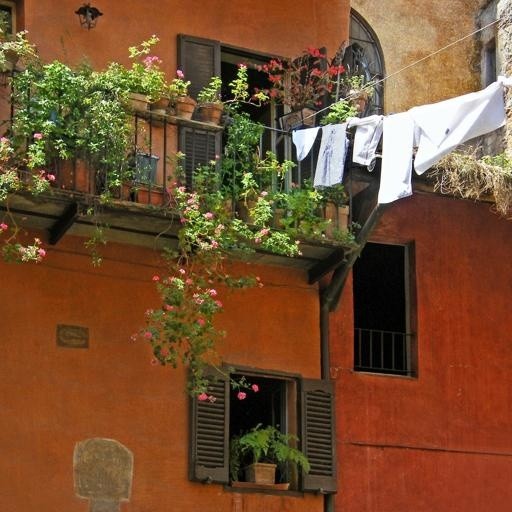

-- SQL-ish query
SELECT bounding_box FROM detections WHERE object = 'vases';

[277,110,317,133]
[111,150,164,203]
[147,95,196,121]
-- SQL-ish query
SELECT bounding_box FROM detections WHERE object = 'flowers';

[236,46,345,112]
[145,57,196,98]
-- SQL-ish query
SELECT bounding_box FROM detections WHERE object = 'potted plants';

[199,63,268,124]
[302,177,351,240]
[265,189,288,229]
[50,103,96,194]
[235,422,309,486]
[341,72,379,113]
[124,34,156,110]
[237,169,263,227]
